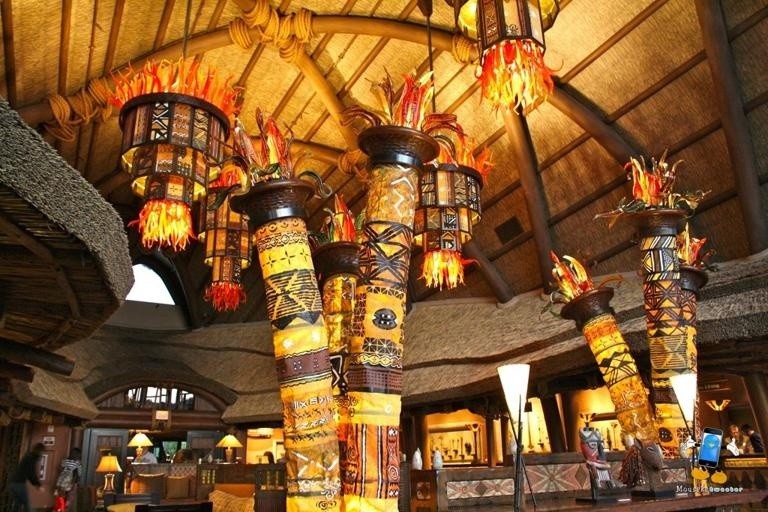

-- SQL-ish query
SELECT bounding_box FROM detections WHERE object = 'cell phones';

[698,426,724,469]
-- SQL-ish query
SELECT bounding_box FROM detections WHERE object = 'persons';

[740,424,766,453]
[134,446,158,464]
[263,451,276,465]
[277,451,287,463]
[51,447,84,511]
[724,424,756,454]
[8,442,47,511]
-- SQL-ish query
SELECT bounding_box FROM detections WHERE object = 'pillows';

[166,475,189,499]
[131,472,165,499]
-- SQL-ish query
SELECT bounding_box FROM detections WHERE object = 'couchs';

[125,463,197,506]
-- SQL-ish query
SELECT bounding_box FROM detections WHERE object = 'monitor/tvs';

[191,448,213,464]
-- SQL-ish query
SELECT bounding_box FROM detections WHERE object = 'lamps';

[119,0,237,251]
[410,1,484,294]
[667,373,699,471]
[96,452,122,492]
[217,433,243,463]
[127,432,153,462]
[204,180,257,313]
[498,362,540,512]
[446,0,563,120]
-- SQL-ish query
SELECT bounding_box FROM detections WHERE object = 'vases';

[412,447,422,470]
[433,451,442,469]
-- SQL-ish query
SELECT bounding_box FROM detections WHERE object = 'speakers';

[525,402,532,412]
